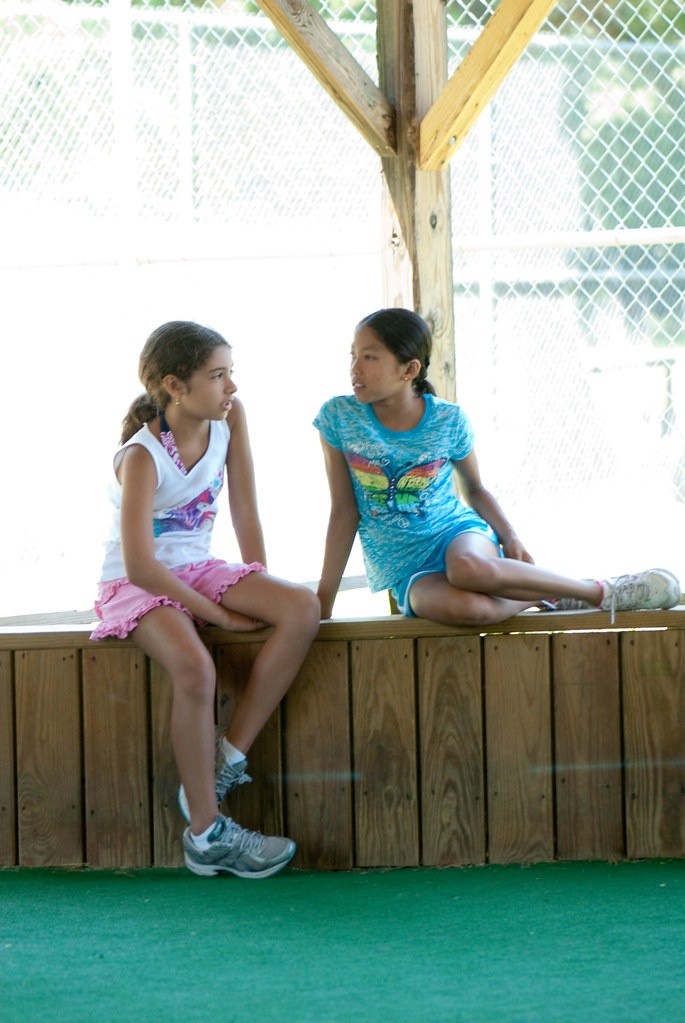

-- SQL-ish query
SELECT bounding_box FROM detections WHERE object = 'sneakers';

[182,813,296,879]
[177,730,252,823]
[537,575,596,612]
[595,568,682,625]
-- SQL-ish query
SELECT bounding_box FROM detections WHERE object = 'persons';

[314,308,681,623]
[91,320,321,877]
